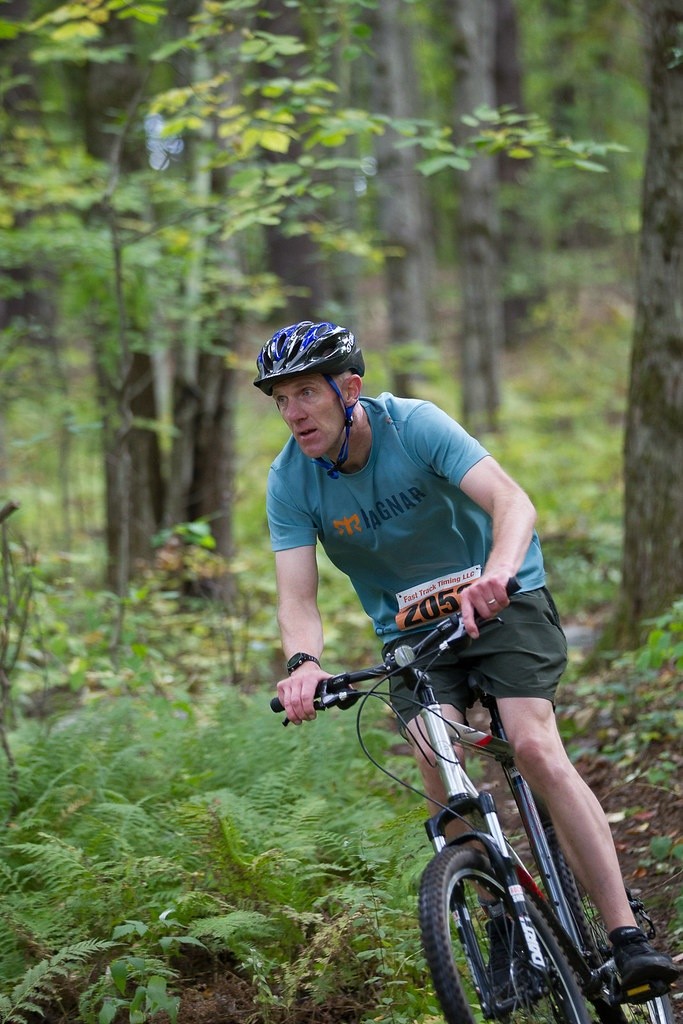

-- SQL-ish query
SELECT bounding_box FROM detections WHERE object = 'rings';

[486,598,496,604]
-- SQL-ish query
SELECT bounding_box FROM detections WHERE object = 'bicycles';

[269,577,676,1024]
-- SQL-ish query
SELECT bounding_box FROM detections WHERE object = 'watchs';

[286,652,321,677]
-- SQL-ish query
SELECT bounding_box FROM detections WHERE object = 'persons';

[252,323,683,994]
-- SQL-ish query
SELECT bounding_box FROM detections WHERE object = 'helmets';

[253,319,365,397]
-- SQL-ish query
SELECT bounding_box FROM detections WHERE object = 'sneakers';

[485,912,525,980]
[608,926,679,985]
